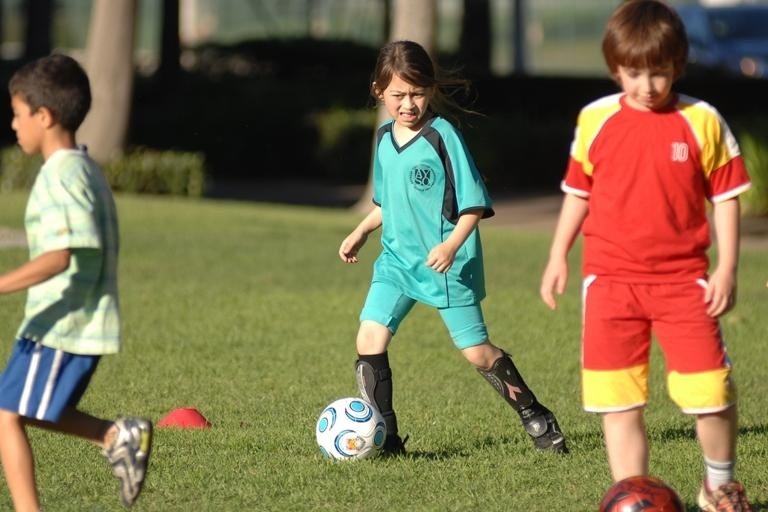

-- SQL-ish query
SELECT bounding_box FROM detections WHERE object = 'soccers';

[314,397,386,464]
[598,474,684,511]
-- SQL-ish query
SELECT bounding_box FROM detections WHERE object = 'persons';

[338,41,567,461]
[0,54,151,512]
[540,0,752,512]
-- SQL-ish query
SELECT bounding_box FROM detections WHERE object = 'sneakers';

[696,472,752,512]
[365,435,405,460]
[100,415,152,508]
[525,412,568,455]
